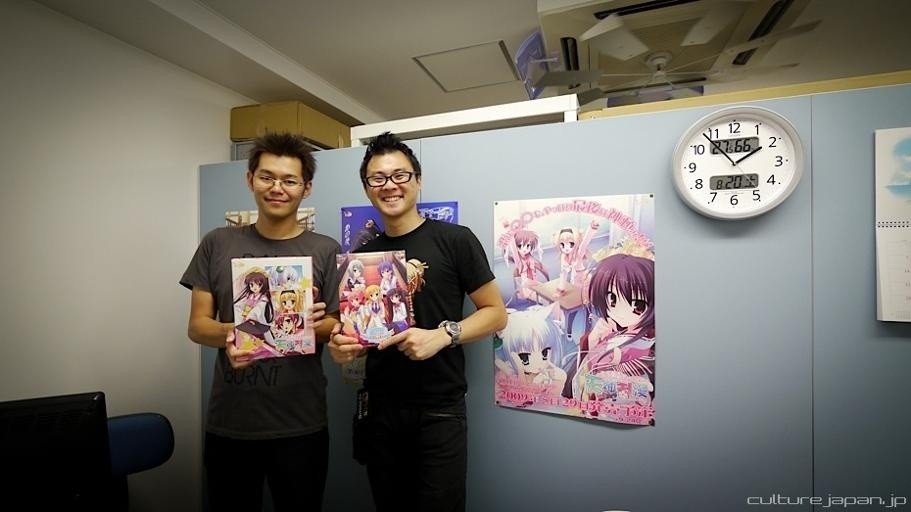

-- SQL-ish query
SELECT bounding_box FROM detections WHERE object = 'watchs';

[437,319,461,349]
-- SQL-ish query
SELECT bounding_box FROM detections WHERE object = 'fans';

[524,0,823,108]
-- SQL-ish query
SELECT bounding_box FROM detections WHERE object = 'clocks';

[671,105,804,221]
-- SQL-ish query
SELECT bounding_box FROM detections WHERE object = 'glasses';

[366,172,412,187]
[255,176,305,191]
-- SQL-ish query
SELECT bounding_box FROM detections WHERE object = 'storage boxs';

[229,101,350,150]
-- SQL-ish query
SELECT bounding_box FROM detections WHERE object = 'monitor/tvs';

[0,391,123,511]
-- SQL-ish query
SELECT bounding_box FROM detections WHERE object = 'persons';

[327,131,511,511]
[178,132,349,511]
[494,216,655,426]
[341,260,408,345]
[231,264,306,356]
